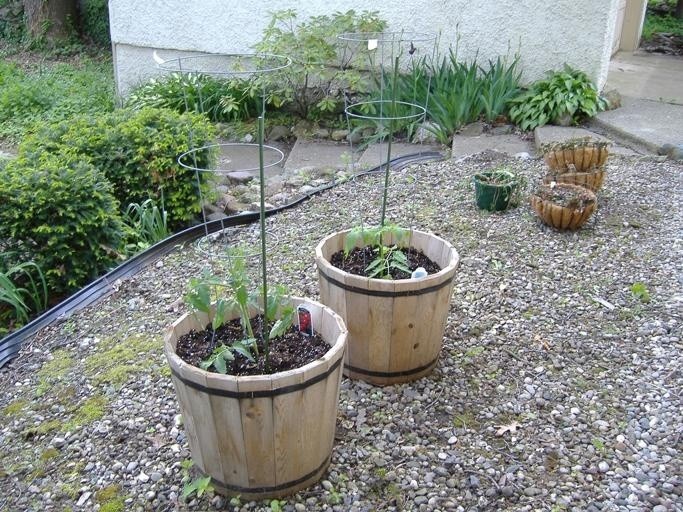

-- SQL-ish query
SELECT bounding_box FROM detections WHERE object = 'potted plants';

[505,62,605,130]
[469,166,527,213]
[316,217,459,386]
[159,250,349,500]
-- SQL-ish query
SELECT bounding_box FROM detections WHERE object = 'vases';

[544,167,605,195]
[530,184,598,228]
[544,140,608,172]
